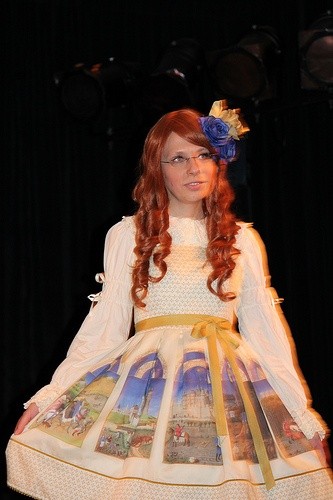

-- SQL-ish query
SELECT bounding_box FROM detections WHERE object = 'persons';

[6,99,333,499]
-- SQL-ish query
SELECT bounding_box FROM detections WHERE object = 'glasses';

[160,152,213,169]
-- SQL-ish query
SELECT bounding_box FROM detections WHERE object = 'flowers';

[198,98,252,164]
